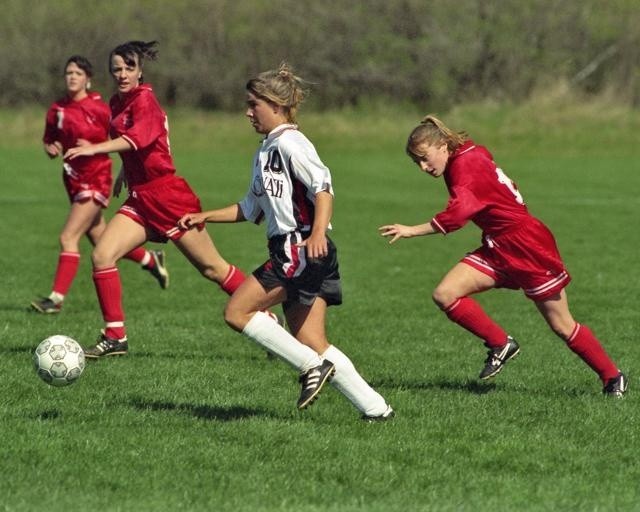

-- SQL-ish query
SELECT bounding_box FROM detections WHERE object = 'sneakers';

[479,335,520,380]
[84,329,128,358]
[297,359,335,409]
[31,297,62,313]
[149,251,169,289]
[602,372,628,398]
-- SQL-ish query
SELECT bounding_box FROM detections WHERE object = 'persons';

[64,40,285,361]
[377,115,629,403]
[177,60,397,423]
[30,55,168,314]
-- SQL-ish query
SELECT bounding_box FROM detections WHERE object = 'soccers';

[34,334,85,386]
